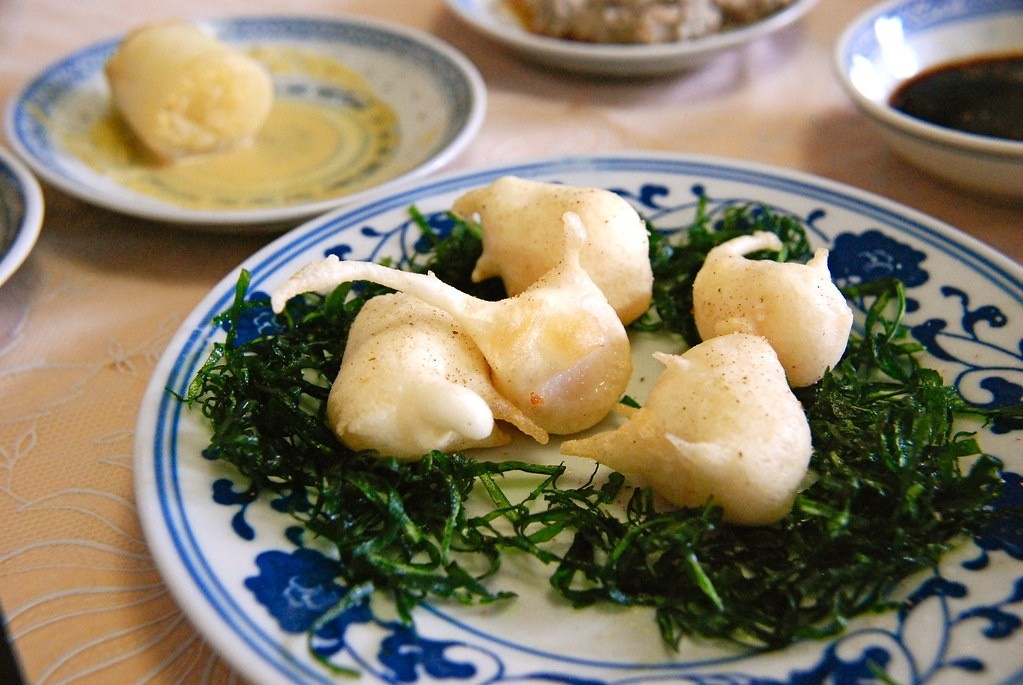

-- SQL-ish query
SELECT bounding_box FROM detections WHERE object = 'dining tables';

[0,0,1023,684]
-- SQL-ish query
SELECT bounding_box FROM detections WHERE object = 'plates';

[829,0,1023,203]
[446,0,819,79]
[131,154,1023,685]
[0,143,45,290]
[7,10,488,236]
